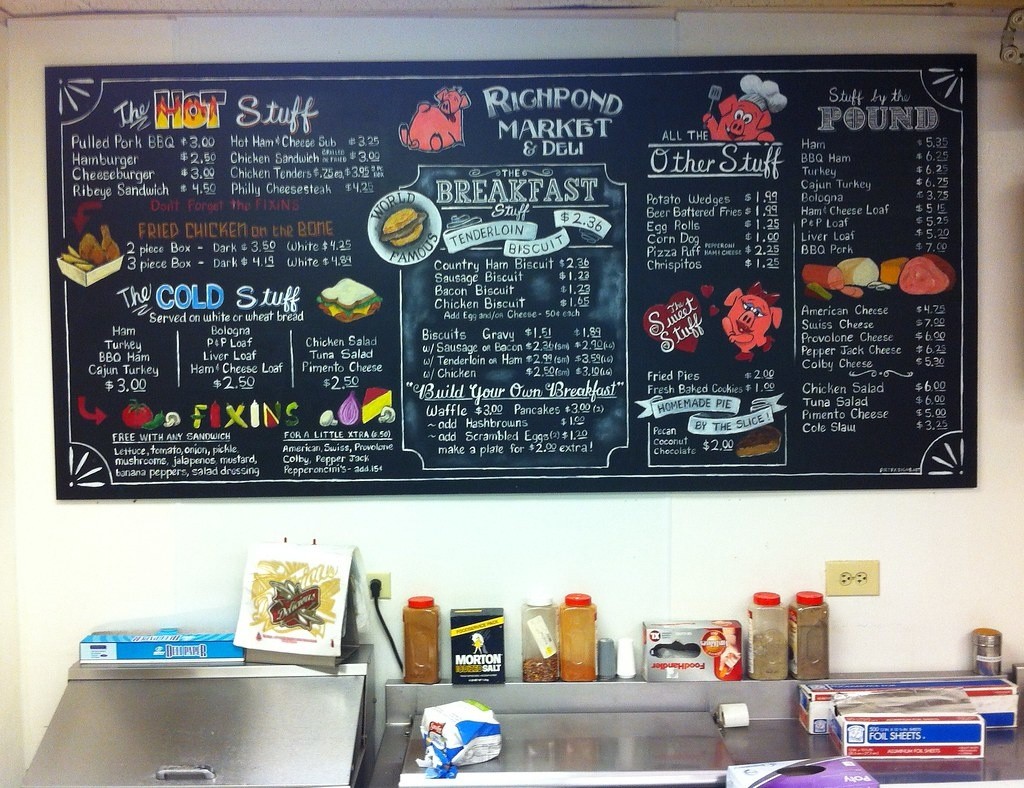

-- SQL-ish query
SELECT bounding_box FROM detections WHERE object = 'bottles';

[403,596,440,685]
[522,596,558,682]
[747,592,788,680]
[973,628,1002,675]
[598,638,636,681]
[558,593,597,683]
[788,592,828,679]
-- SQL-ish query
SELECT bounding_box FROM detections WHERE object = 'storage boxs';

[451,608,505,684]
[79,635,245,663]
[725,756,879,788]
[641,620,742,682]
[795,678,1019,758]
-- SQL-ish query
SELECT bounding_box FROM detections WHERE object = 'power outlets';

[367,573,391,599]
[824,560,879,596]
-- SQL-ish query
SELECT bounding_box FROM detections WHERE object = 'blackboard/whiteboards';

[42,55,978,500]
